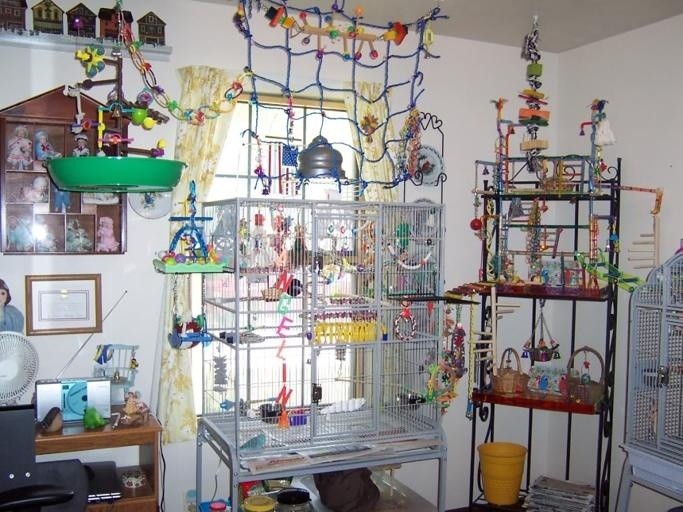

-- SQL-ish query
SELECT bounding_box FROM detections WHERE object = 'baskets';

[541,160,576,193]
[566,347,604,405]
[491,348,529,393]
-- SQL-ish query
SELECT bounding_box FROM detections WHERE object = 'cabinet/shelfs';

[469,153,622,511]
[616,249,683,511]
[195,199,447,511]
[0,85,130,253]
[35,403,165,512]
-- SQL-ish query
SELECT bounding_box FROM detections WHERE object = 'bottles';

[243,496,276,512]
[209,502,227,512]
[275,487,314,512]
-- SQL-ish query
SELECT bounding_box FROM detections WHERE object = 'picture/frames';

[25,273,103,336]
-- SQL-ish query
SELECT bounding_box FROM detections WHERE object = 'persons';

[3,124,123,252]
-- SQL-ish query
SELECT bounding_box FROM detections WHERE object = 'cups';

[476,441,527,506]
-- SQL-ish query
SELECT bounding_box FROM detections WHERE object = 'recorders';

[33,376,111,425]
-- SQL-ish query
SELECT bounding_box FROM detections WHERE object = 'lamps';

[295,133,348,180]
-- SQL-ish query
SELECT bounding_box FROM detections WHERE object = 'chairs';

[0,407,89,512]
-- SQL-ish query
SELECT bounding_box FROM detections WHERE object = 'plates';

[129,189,176,220]
[409,199,442,241]
[408,146,444,185]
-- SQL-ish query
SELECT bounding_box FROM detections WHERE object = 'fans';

[0,331,40,407]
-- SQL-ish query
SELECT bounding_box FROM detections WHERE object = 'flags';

[261,138,301,196]
